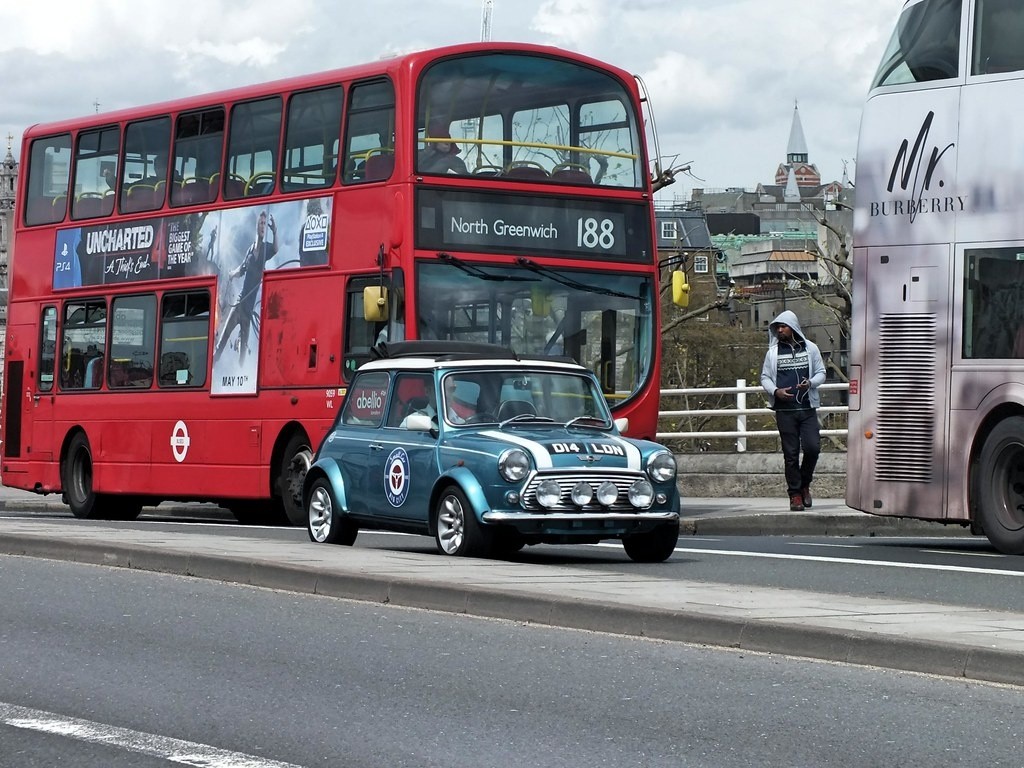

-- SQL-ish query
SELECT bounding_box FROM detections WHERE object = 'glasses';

[447,384,458,393]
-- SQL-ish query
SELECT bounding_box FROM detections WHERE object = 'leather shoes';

[789,495,805,511]
[802,486,812,507]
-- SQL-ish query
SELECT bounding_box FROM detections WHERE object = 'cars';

[301,340,682,566]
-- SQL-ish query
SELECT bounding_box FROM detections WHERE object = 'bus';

[845,0,1024,557]
[0,43,691,530]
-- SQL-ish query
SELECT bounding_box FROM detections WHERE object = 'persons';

[399,374,465,428]
[103,153,185,192]
[74,341,104,388]
[761,310,826,511]
[417,122,470,174]
[375,300,439,347]
[213,211,279,367]
[356,142,395,180]
[205,225,217,261]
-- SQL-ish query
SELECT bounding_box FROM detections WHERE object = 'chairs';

[126,184,155,212]
[493,400,538,423]
[101,189,125,214]
[506,162,548,180]
[365,148,395,180]
[253,177,275,195]
[401,397,431,419]
[552,163,594,185]
[181,176,210,202]
[208,171,246,200]
[243,171,276,196]
[51,195,76,221]
[154,180,182,208]
[84,356,124,388]
[77,192,103,217]
[472,164,503,176]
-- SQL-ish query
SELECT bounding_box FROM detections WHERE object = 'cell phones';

[801,380,806,384]
